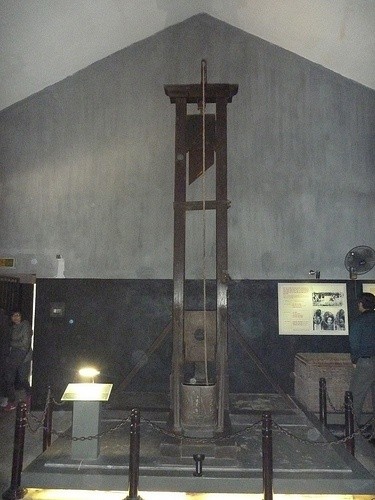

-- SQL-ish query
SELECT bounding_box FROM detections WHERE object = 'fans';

[343,245,375,280]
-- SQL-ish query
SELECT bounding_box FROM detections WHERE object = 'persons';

[4,311,32,412]
[313,292,345,331]
[333,293,375,445]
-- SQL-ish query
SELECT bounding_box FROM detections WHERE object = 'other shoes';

[3,402,16,411]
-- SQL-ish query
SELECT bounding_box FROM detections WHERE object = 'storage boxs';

[293,352,375,415]
